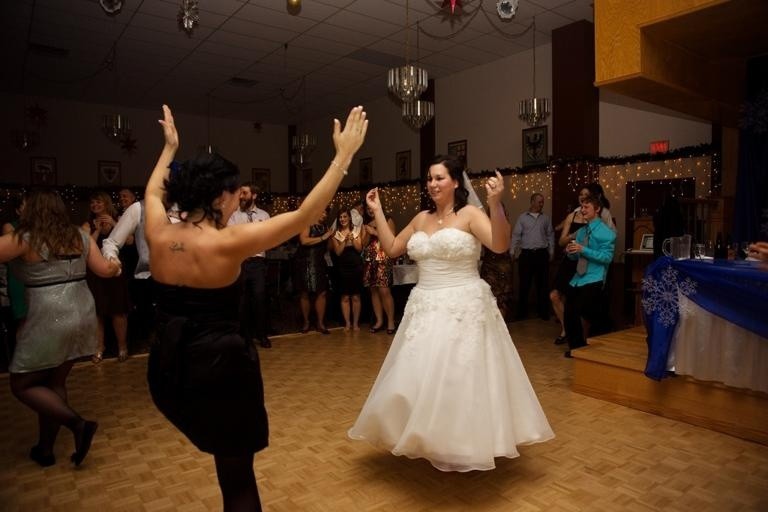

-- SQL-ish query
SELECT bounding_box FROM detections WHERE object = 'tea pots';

[662,234,693,261]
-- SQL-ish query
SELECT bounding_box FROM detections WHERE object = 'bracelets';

[330,159,351,179]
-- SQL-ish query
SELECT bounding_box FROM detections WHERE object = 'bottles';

[570,237,576,255]
[716,231,722,259]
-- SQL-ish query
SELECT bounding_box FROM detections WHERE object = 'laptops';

[631,234,654,253]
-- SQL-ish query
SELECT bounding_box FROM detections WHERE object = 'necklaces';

[438,203,453,225]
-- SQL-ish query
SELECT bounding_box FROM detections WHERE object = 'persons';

[362,155,556,471]
[747,241,768,265]
[295,204,395,335]
[142,104,370,512]
[3,178,272,468]
[480,182,618,359]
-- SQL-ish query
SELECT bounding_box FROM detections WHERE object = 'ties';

[576,233,589,276]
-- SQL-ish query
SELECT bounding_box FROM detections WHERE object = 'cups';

[694,243,706,259]
[705,240,713,248]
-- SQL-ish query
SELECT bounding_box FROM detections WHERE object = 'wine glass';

[741,241,751,260]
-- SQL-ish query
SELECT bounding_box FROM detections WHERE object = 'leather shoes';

[555,336,566,344]
[71,421,97,465]
[301,326,330,334]
[260,335,271,348]
[30,446,56,466]
[344,324,361,332]
[371,322,395,334]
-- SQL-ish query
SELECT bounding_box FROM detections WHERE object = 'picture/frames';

[356,158,373,186]
[395,150,412,182]
[447,142,468,171]
[252,168,270,192]
[97,159,121,188]
[518,128,550,169]
[30,156,58,186]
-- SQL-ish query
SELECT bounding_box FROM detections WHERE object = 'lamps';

[101,76,134,142]
[11,90,42,152]
[400,1,548,133]
[381,3,429,98]
[292,130,325,174]
